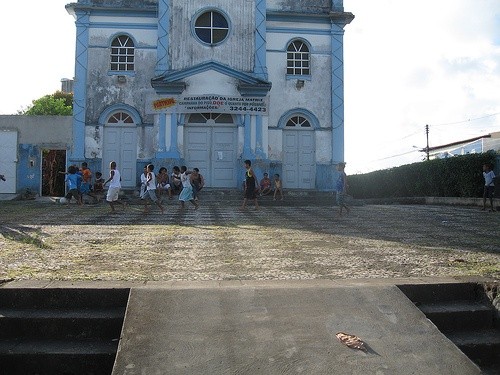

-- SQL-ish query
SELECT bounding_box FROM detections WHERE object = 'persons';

[102,162,126,213]
[178,166,200,210]
[157,167,172,200]
[145,164,164,210]
[481,164,496,211]
[273,174,283,200]
[94,171,103,193]
[139,166,147,200]
[58,165,84,208]
[79,162,99,201]
[259,173,272,196]
[191,168,204,200]
[171,166,182,194]
[243,160,260,208]
[335,162,350,215]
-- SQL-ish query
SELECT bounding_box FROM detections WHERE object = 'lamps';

[296,80,304,89]
[118,76,126,83]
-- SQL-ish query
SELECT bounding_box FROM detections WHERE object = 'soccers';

[60,198,66,204]
